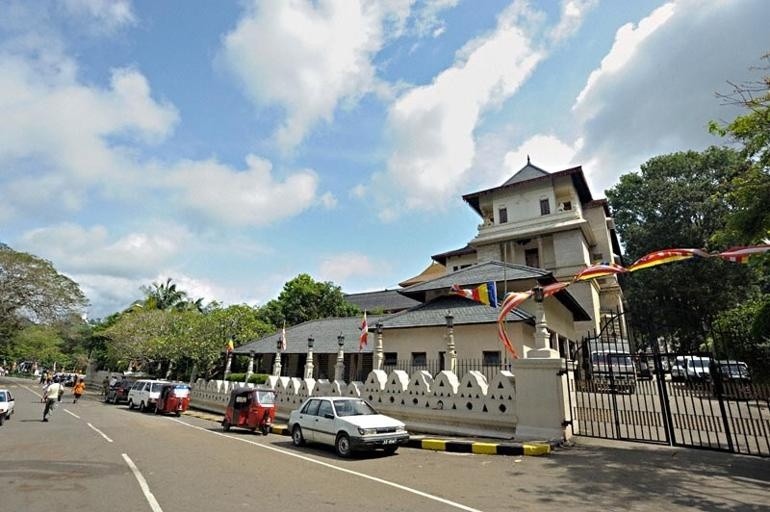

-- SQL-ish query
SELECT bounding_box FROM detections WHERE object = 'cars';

[0,390,16,426]
[283,394,409,460]
[103,380,134,405]
[672,352,753,398]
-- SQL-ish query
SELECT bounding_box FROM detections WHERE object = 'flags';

[280,326,286,350]
[453,280,498,308]
[359,310,368,351]
[227,332,234,353]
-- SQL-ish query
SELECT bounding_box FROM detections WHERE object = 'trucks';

[591,350,636,393]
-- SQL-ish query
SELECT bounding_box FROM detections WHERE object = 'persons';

[121,375,127,388]
[101,375,108,395]
[110,375,116,385]
[39,369,85,421]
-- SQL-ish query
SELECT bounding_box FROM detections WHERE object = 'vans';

[126,378,173,413]
